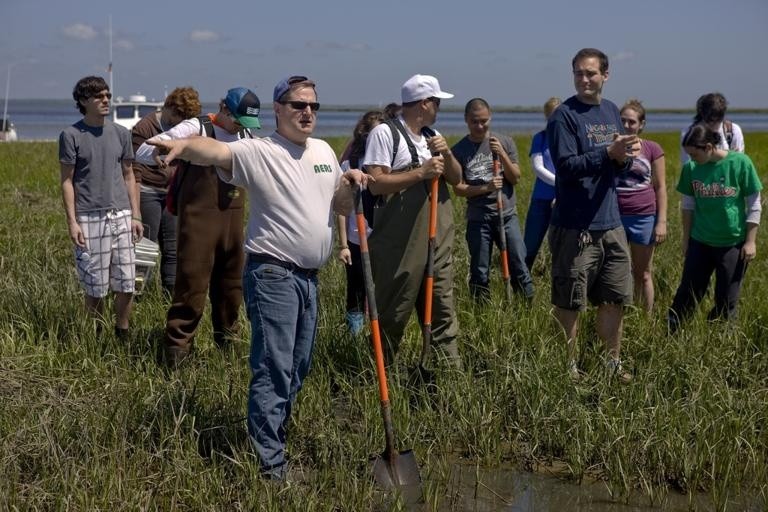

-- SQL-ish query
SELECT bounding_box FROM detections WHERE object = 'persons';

[137,88,262,366]
[664,123,764,337]
[337,103,403,337]
[450,98,534,308]
[548,48,642,384]
[680,92,744,170]
[59,75,143,349]
[524,97,561,273]
[145,75,376,486]
[614,99,667,320]
[130,87,203,304]
[363,75,462,373]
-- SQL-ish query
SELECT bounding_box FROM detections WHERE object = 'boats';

[107,16,174,132]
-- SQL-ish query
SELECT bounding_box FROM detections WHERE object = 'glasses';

[464,117,492,127]
[93,90,113,100]
[230,116,240,127]
[285,99,322,111]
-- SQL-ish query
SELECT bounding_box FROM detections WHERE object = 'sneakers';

[615,365,632,384]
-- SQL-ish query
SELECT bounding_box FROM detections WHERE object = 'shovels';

[408,153,439,414]
[350,179,421,504]
[492,151,522,322]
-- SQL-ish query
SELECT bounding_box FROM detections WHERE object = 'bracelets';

[340,246,349,248]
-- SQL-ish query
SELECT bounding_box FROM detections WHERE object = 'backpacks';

[164,117,245,216]
[361,122,439,228]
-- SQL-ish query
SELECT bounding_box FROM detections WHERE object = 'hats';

[400,73,455,105]
[271,73,308,99]
[224,86,262,129]
